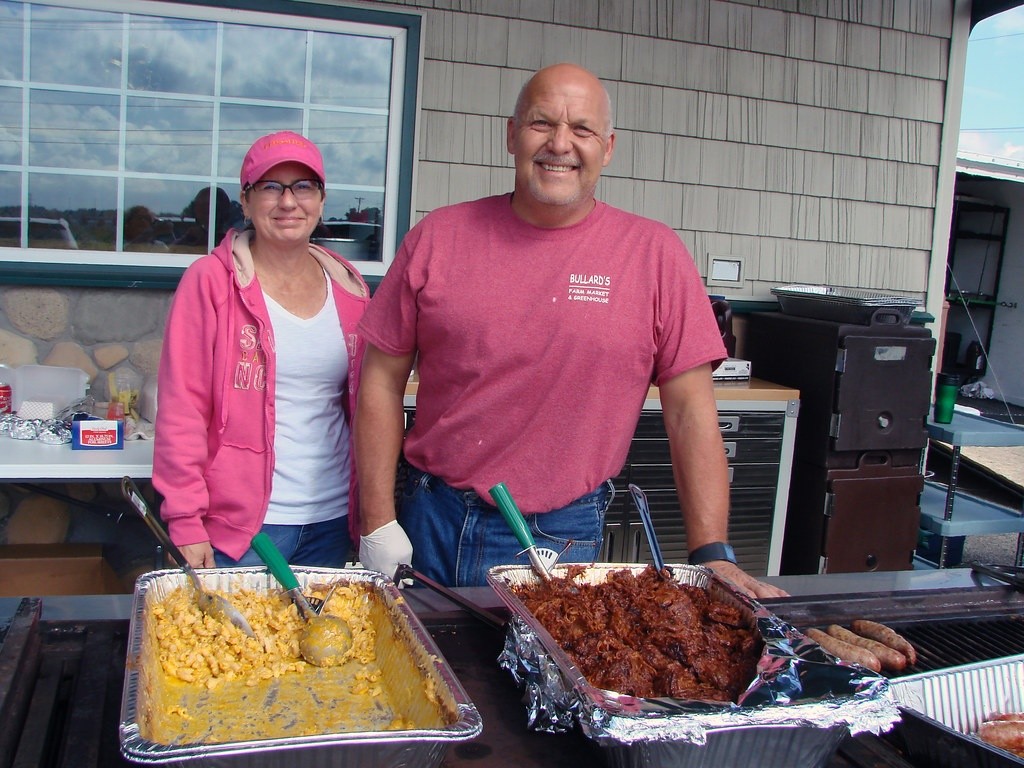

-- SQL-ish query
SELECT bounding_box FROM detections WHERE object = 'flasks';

[934,371,958,424]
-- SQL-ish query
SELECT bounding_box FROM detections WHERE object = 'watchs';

[687,541,738,566]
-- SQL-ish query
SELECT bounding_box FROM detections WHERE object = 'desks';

[0,569,1023,768]
[0,423,164,570]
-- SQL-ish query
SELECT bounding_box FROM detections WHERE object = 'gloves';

[360,517,417,590]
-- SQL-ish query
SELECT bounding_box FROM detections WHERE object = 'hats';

[238,132,327,193]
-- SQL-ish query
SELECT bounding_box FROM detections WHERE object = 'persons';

[150,131,372,570]
[352,63,792,601]
[122,186,233,248]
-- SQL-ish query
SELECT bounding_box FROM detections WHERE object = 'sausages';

[978,711,1023,759]
[807,618,917,672]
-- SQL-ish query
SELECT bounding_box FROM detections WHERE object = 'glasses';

[239,177,325,198]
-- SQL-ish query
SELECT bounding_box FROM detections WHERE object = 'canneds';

[0,383,12,413]
